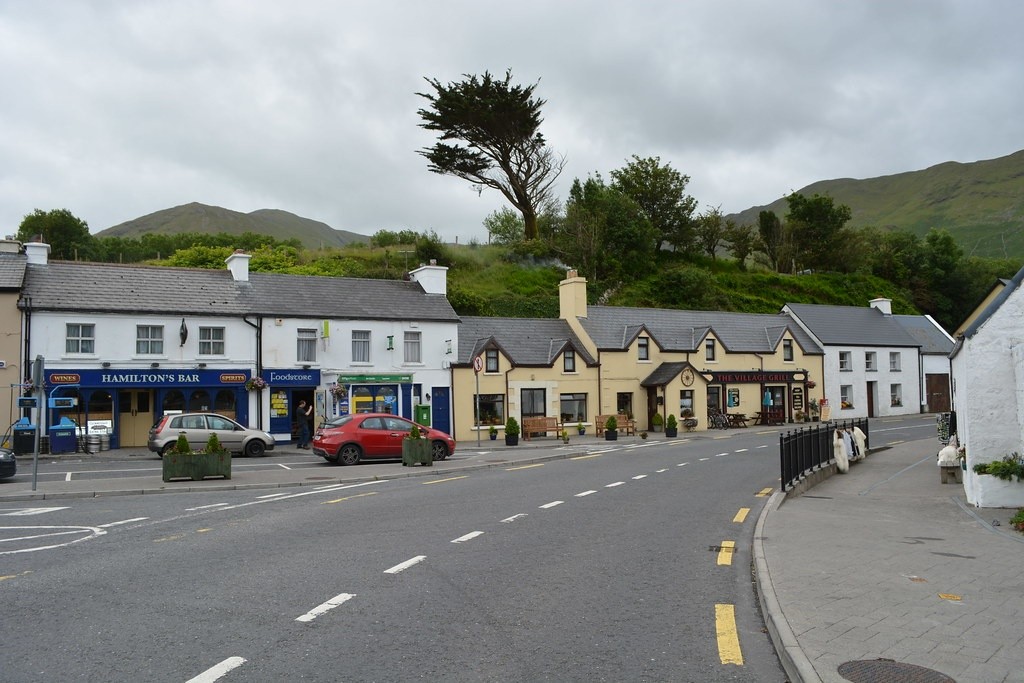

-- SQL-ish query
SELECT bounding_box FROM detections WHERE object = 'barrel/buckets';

[101,434,110,450]
[87,435,101,453]
[39,437,50,454]
[76,436,86,452]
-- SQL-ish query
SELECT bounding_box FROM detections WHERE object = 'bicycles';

[706,405,731,430]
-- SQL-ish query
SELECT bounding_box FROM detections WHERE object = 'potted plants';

[401,425,433,467]
[639,431,649,440]
[972,453,1024,507]
[796,410,808,424]
[604,415,618,441]
[681,409,694,420]
[652,413,663,433]
[809,398,820,422]
[488,427,499,441]
[806,381,816,389]
[665,414,678,437]
[504,416,521,446]
[162,433,232,482]
[577,423,586,435]
[561,429,569,444]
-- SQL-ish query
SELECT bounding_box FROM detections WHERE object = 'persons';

[297,401,312,450]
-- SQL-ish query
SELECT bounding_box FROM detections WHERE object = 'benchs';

[594,415,635,438]
[523,417,564,440]
[937,451,962,483]
[727,418,749,429]
[751,416,783,425]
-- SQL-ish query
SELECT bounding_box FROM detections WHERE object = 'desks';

[754,412,783,426]
[724,413,750,428]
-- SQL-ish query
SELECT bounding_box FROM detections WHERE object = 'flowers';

[245,377,269,392]
[330,384,347,402]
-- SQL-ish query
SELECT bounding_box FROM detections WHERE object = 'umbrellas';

[762,388,771,424]
[728,388,734,407]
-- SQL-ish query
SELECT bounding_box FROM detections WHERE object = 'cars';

[311,413,456,467]
[147,412,276,458]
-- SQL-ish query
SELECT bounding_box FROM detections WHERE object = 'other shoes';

[304,446,310,449]
[297,445,303,447]
